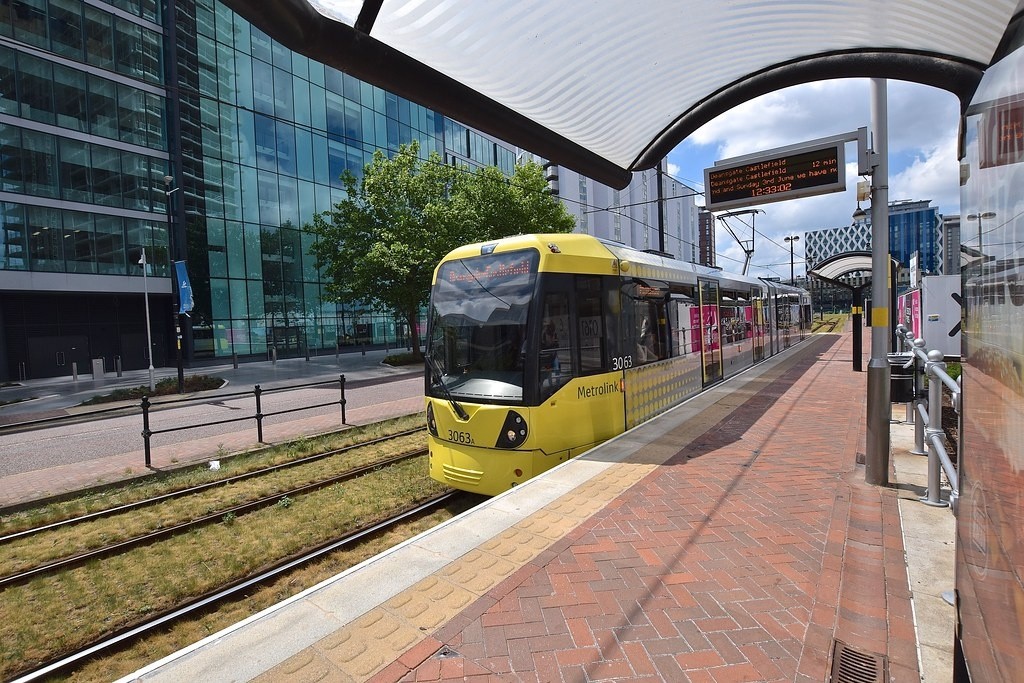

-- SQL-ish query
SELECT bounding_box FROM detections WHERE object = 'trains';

[423,209,814,497]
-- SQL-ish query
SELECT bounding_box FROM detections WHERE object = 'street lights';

[784,234,799,288]
[967,211,996,316]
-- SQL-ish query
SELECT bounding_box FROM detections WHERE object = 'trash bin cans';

[887,352,914,403]
